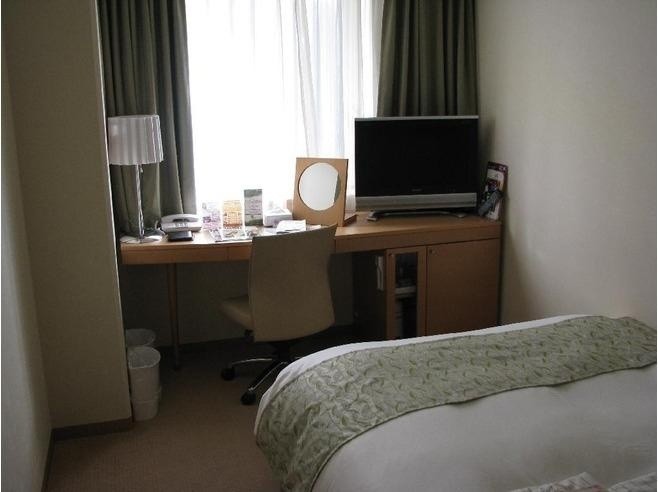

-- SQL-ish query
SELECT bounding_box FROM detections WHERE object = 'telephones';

[161,214,203,232]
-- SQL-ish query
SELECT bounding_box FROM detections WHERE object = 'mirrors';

[292,158,348,227]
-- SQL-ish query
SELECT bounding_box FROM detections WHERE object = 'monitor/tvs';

[353,116,480,221]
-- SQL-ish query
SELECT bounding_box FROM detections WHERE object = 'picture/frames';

[477,161,508,220]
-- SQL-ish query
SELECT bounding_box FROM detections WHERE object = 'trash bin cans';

[125,328,161,422]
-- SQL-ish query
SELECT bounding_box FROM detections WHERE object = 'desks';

[116,212,501,340]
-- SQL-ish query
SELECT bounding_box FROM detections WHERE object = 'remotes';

[478,188,503,216]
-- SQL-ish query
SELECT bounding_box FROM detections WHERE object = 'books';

[167,230,192,241]
[276,219,307,234]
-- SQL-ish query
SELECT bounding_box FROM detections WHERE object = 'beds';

[253,313,655,491]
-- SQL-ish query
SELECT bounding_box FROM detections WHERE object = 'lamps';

[107,113,165,243]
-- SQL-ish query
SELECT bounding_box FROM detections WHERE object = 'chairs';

[224,220,339,407]
